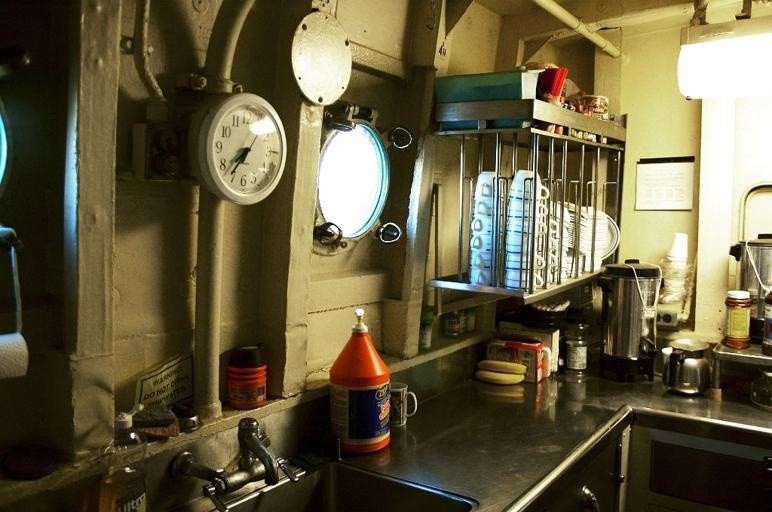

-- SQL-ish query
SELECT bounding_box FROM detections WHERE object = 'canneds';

[566,323,590,379]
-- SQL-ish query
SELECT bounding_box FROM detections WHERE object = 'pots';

[728,231,771,331]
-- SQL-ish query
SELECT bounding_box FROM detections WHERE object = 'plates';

[568,203,620,272]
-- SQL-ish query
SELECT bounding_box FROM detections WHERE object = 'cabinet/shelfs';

[427,99,625,305]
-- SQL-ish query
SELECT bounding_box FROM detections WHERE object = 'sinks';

[208,459,480,512]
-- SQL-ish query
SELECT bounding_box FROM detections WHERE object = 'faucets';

[237,417,279,486]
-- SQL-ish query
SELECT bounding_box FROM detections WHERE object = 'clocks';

[204,93,287,206]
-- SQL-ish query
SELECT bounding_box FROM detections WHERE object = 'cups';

[664,231,689,298]
[660,345,672,365]
[467,169,557,293]
[389,381,418,425]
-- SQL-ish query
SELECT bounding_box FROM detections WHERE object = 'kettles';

[603,258,662,386]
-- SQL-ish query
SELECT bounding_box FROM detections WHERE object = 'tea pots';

[667,338,713,396]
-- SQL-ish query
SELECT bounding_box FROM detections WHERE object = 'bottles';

[564,320,589,376]
[760,287,772,356]
[100,402,149,512]
[419,308,477,350]
[565,368,589,418]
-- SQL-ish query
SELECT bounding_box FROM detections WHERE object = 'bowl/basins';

[548,200,570,282]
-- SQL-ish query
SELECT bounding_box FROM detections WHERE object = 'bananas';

[475,360,527,385]
